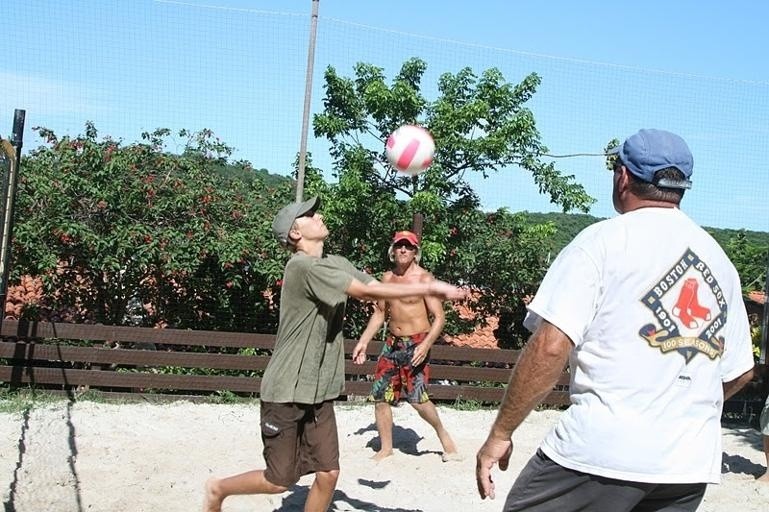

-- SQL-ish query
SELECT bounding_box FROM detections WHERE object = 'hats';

[393,230,419,247]
[270,195,321,247]
[606,127,694,191]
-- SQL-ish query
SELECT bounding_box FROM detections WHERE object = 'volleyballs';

[386,125,435,173]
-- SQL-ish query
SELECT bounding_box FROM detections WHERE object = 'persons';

[477,127,756,510]
[204,193,471,511]
[352,231,463,461]
